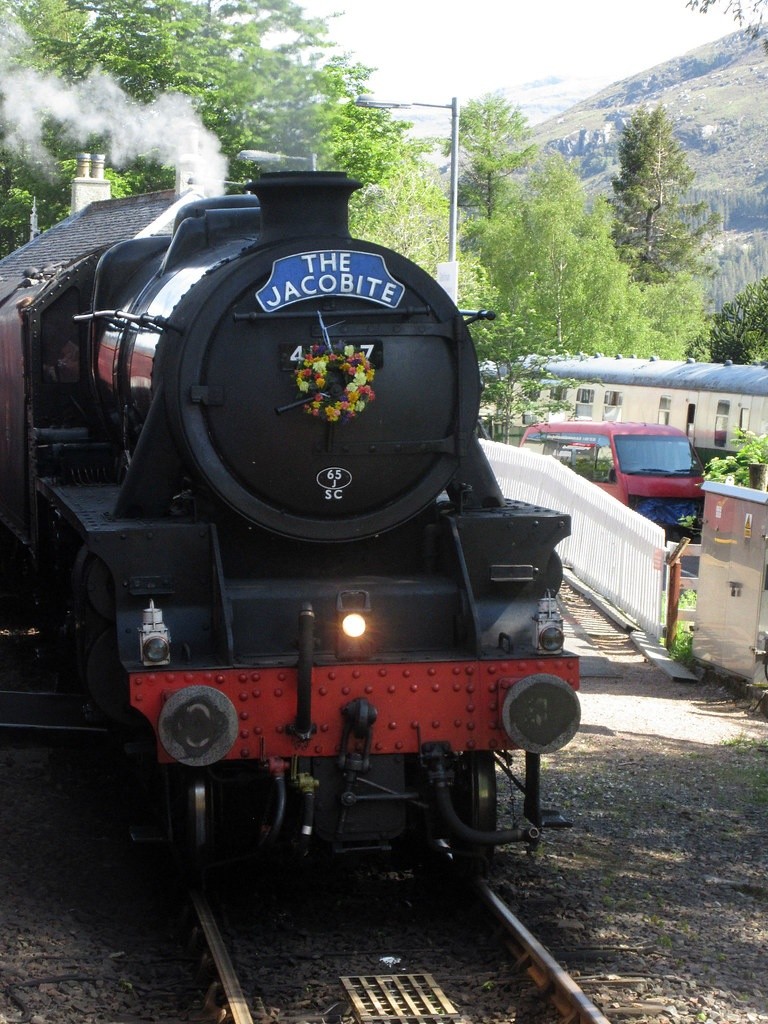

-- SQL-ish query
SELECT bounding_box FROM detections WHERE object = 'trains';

[0,169,585,865]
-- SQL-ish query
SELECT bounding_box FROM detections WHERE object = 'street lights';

[355,88,466,259]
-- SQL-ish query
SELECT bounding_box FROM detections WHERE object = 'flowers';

[298,345,376,420]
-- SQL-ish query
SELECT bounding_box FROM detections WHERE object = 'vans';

[520,420,707,510]
